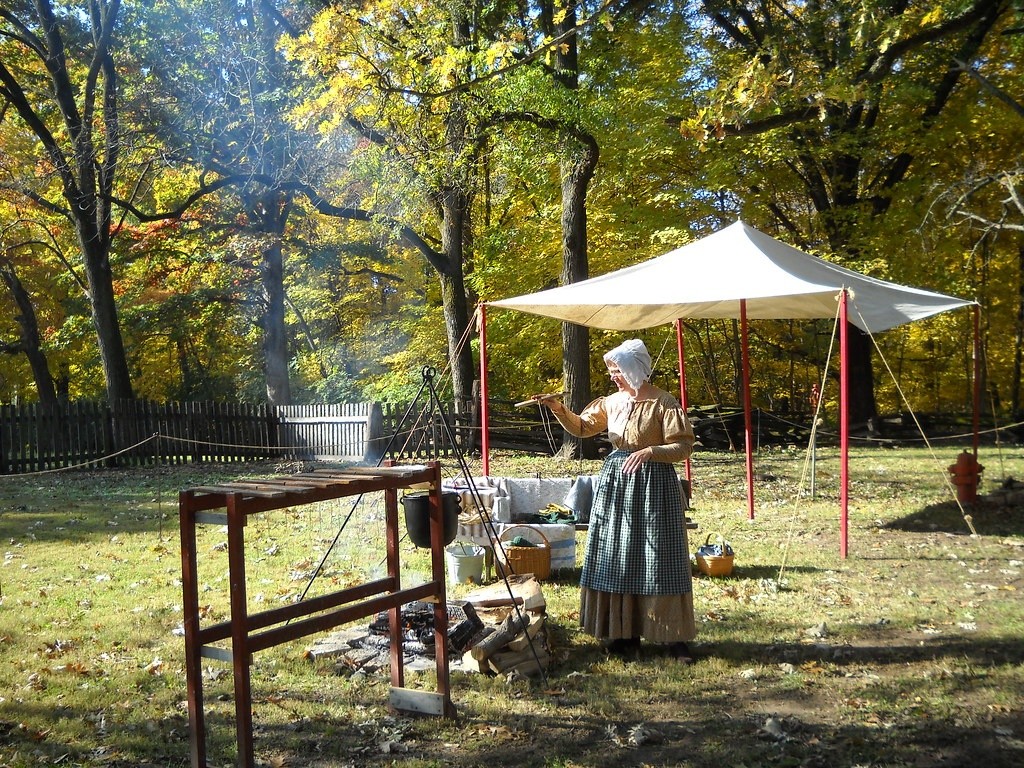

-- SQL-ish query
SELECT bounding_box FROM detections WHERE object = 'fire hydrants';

[947,449,985,503]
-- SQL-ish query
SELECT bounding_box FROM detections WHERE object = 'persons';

[531,338,697,652]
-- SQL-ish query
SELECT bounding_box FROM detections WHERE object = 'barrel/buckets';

[445,545,486,587]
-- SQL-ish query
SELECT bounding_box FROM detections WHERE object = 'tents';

[477,218,985,557]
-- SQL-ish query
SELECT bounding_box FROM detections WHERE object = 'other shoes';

[608,636,640,652]
[670,642,695,663]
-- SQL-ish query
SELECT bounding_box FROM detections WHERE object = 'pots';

[400,464,462,549]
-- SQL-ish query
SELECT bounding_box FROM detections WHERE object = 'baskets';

[696,533,734,576]
[495,526,551,580]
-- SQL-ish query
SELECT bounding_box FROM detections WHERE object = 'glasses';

[609,368,623,374]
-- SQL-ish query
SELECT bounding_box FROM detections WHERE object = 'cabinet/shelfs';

[180,459,449,767]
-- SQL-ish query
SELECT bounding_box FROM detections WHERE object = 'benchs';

[442,475,698,586]
